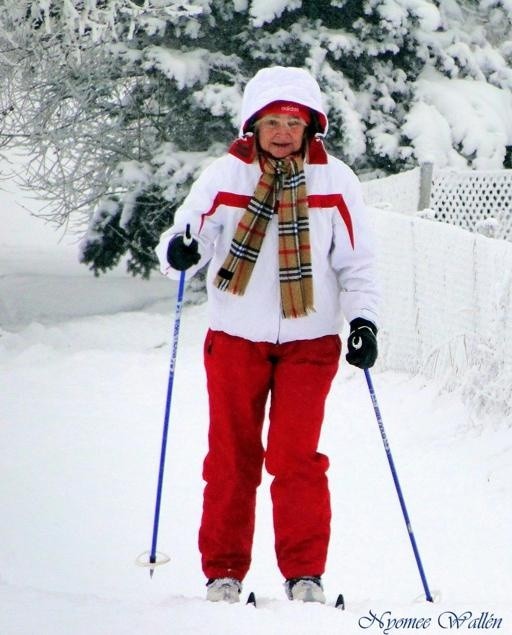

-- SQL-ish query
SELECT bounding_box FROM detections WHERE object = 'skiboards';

[244,591,346,611]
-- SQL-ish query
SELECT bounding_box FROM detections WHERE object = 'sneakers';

[286,576,326,603]
[207,577,241,603]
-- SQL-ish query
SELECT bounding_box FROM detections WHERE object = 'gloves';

[346,318,377,368]
[167,236,200,270]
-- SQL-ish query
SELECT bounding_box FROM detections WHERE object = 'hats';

[256,102,311,123]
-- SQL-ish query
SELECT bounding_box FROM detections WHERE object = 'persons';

[153,65,381,604]
